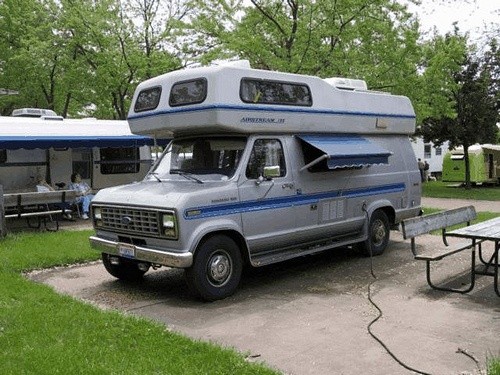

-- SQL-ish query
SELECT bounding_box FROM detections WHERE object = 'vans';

[89,60,424,303]
[439,144,500,188]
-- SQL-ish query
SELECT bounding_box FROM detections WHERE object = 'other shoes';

[82,213,89,220]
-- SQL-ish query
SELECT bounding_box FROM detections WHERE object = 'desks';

[444,216,500,296]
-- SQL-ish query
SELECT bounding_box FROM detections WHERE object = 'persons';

[35,176,53,192]
[417,157,436,183]
[70,171,94,220]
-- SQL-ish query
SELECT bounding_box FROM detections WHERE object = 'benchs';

[3,190,76,231]
[401,205,500,296]
